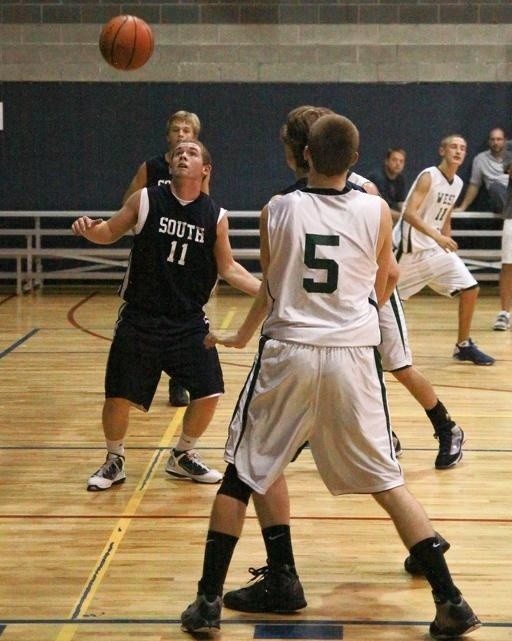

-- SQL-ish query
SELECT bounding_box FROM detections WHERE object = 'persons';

[392,134,495,367]
[492,139,512,332]
[452,123,512,215]
[367,143,408,229]
[121,109,212,408]
[72,136,262,494]
[179,115,484,640]
[203,102,451,613]
[346,170,466,469]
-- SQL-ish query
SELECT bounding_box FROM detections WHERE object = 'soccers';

[99,15,153,72]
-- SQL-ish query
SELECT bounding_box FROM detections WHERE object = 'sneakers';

[494,315,510,330]
[168,379,189,407]
[453,339,494,366]
[165,448,224,483]
[223,573,307,612]
[87,452,126,491]
[405,534,481,638]
[181,595,221,632]
[392,421,464,468]
